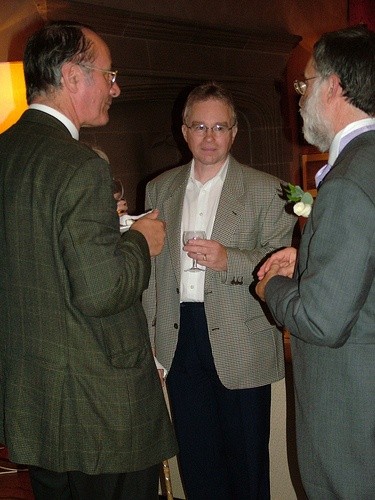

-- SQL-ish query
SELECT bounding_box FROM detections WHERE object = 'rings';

[203,254,206,261]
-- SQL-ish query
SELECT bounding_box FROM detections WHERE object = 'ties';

[315,124,375,188]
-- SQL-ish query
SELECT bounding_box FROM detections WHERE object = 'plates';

[119,209,155,233]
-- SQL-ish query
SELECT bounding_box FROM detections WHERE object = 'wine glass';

[183,231,207,273]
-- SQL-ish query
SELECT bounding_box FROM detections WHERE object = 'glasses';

[75,62,118,84]
[294,76,318,96]
[184,123,237,136]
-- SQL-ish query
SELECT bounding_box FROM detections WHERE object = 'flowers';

[287,180,312,217]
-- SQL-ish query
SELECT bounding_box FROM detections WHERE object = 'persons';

[255,25,375,500]
[142,84,299,500]
[0,20,178,500]
